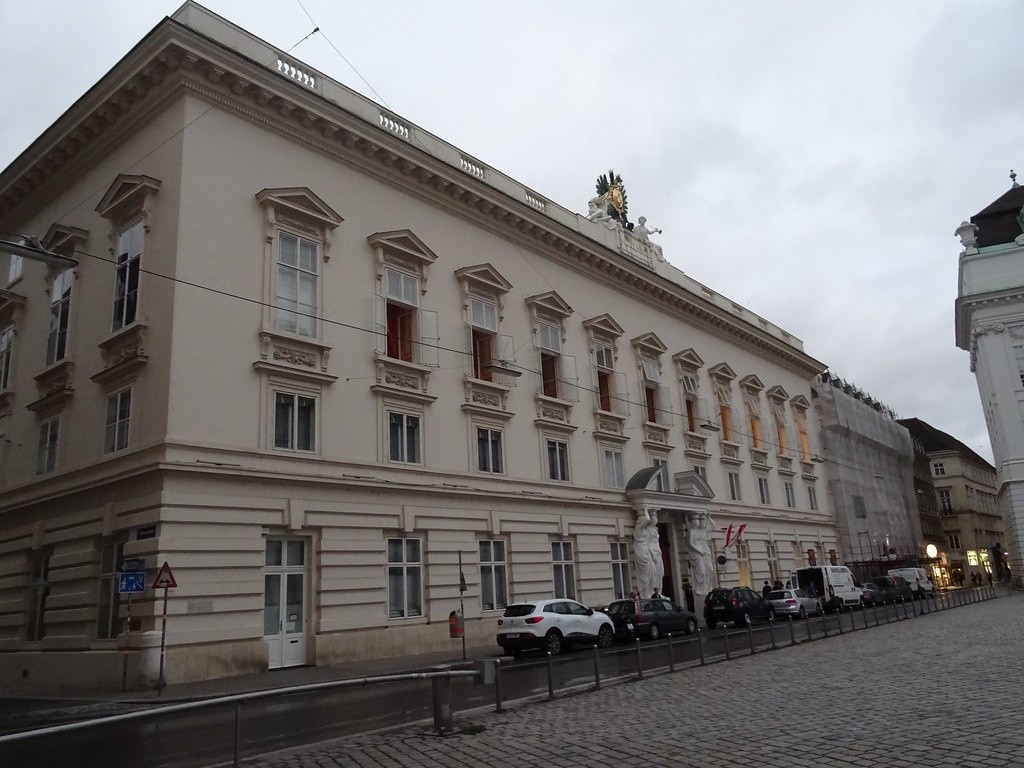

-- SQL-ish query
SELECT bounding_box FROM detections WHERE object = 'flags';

[726,524,746,547]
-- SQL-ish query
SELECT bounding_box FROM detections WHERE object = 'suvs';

[702,585,776,627]
[495,596,615,655]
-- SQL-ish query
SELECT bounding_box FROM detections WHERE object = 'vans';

[888,568,935,599]
[871,575,912,603]
[789,565,867,609]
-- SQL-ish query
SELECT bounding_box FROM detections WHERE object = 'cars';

[858,583,891,605]
[604,599,699,639]
[762,589,822,618]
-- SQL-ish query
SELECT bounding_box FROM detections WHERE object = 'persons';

[634,510,665,599]
[828,585,843,610]
[588,195,611,223]
[634,216,664,262]
[987,572,993,587]
[687,519,714,594]
[651,587,661,598]
[970,572,977,585]
[774,577,784,590]
[976,570,982,585]
[952,570,963,587]
[763,580,772,599]
